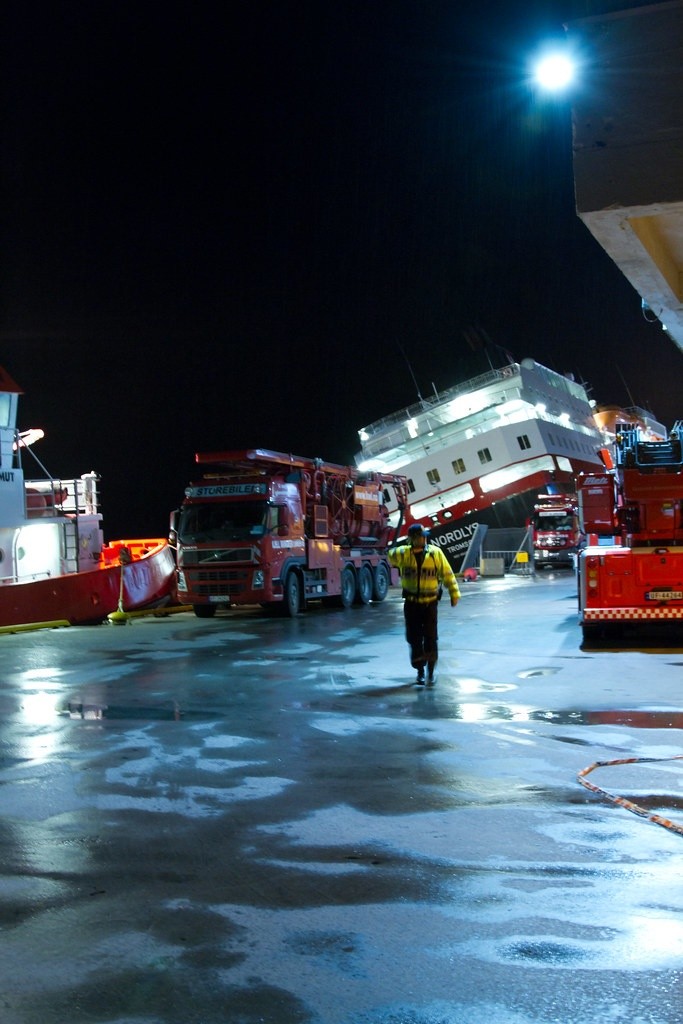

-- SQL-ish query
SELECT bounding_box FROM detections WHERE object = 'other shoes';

[428,669,437,685]
[417,669,426,685]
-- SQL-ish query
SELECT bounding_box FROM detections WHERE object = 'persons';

[388,524,460,687]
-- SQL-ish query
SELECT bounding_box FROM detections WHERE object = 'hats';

[408,523,432,537]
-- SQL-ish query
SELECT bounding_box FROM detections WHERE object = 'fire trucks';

[530,490,586,573]
[578,417,682,649]
[166,446,411,619]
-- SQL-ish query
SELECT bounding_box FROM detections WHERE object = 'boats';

[0,361,179,631]
[352,326,614,580]
